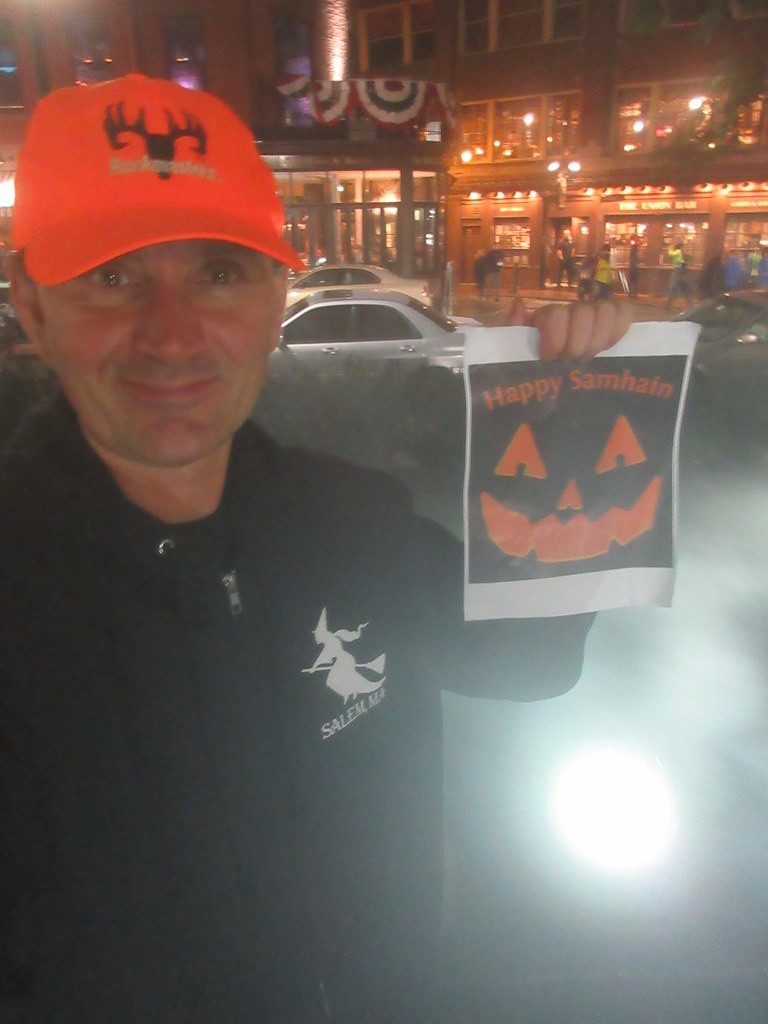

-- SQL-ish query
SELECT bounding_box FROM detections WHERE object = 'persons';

[474,232,768,309]
[0,73,636,1024]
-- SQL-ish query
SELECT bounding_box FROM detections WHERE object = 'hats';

[10,72,306,285]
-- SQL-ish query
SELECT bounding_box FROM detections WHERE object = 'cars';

[285,262,433,308]
[269,292,483,380]
[669,290,766,366]
